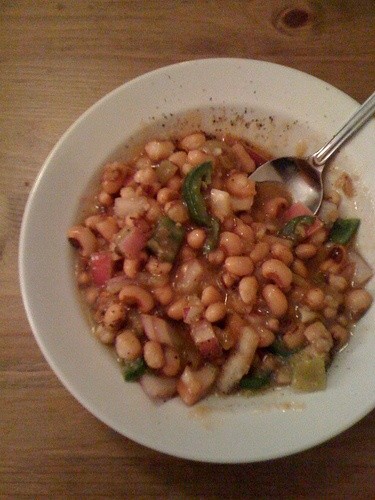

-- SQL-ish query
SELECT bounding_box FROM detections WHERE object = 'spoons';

[247,90,374,216]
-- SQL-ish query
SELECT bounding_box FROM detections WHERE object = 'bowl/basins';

[19,58,374,465]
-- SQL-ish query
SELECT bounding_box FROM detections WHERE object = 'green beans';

[65,134,373,407]
